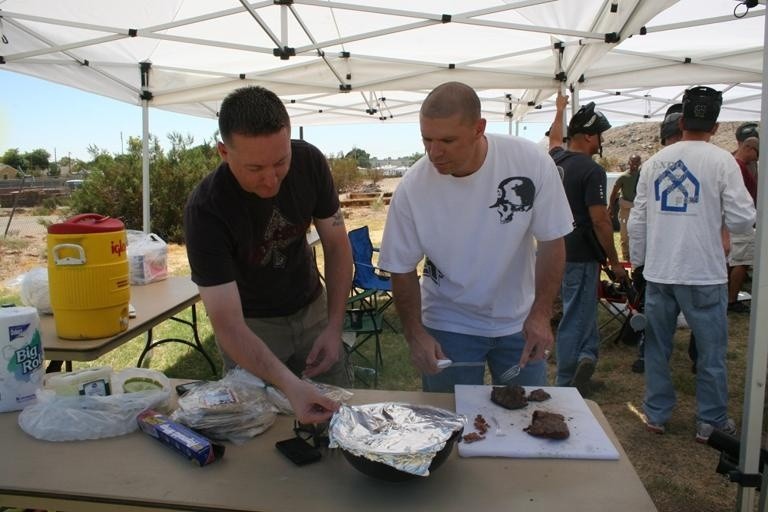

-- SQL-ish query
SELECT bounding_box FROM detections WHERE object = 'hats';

[565,102,612,137]
[683,85,723,122]
[655,101,682,143]
[735,122,758,140]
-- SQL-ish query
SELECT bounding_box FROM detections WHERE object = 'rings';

[542,349,552,360]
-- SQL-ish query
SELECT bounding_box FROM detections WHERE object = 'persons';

[660,102,684,146]
[607,154,642,260]
[722,137,759,314]
[548,91,628,389]
[182,85,355,424]
[731,123,758,183]
[378,82,576,393]
[627,88,757,443]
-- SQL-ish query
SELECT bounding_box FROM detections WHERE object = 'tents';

[0,1,768,511]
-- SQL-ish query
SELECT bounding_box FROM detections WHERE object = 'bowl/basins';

[330,403,462,485]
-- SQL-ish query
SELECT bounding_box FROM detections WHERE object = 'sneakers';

[570,357,597,392]
[647,423,667,436]
[695,419,737,443]
[583,380,606,393]
[632,357,646,373]
[728,301,750,314]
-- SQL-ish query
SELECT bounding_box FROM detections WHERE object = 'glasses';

[746,142,760,157]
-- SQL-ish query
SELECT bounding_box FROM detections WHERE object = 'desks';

[0,371,659,512]
[306,227,325,283]
[39,275,217,376]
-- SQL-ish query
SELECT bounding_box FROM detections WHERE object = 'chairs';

[599,262,641,345]
[344,287,385,389]
[348,226,421,334]
